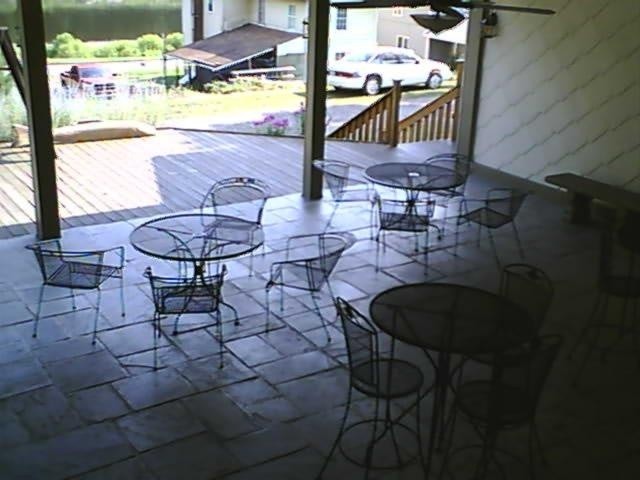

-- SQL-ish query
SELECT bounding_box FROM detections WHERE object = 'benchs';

[544,172,640,234]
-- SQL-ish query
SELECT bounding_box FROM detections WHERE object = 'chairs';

[142,265,227,371]
[24,241,124,345]
[452,187,528,270]
[443,335,562,478]
[571,225,639,389]
[420,155,473,236]
[264,231,357,341]
[436,262,551,455]
[199,177,271,277]
[319,295,425,478]
[370,194,435,278]
[312,159,378,240]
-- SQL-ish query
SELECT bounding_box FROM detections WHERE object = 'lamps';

[480,8,499,39]
[411,3,464,34]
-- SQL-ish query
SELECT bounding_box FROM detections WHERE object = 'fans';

[328,0,558,35]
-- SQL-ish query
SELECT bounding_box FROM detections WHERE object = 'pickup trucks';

[58,62,121,102]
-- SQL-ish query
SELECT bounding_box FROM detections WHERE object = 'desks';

[616,223,639,344]
[130,214,265,335]
[365,164,465,249]
[369,282,532,480]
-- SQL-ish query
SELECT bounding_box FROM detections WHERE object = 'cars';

[324,43,456,97]
